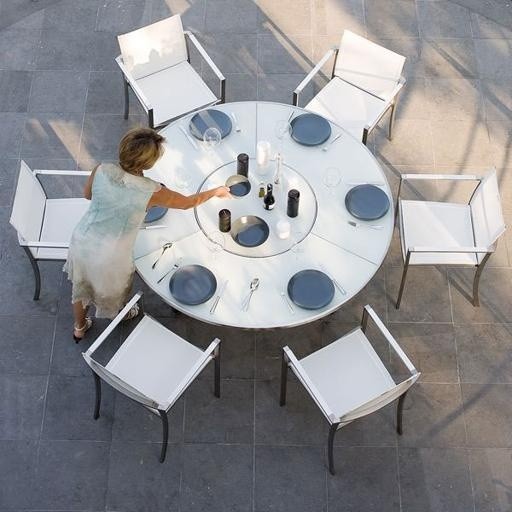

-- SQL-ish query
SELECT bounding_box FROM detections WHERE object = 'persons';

[60,119,232,344]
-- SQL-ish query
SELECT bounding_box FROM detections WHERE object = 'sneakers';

[119,301,140,321]
[72,316,93,344]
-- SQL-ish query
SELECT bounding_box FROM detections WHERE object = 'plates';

[169,265,218,306]
[287,269,336,309]
[228,214,269,249]
[346,184,391,221]
[143,207,167,222]
[291,112,332,147]
[186,110,231,142]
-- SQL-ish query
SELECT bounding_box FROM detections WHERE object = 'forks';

[343,221,387,231]
[321,131,342,153]
[156,257,185,285]
[277,281,296,316]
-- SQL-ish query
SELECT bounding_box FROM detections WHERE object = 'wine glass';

[174,169,193,214]
[205,232,224,264]
[322,166,342,201]
[290,230,308,269]
[204,127,222,154]
[274,119,292,154]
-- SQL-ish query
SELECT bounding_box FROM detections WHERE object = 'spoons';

[151,243,172,270]
[240,278,260,307]
[228,111,244,137]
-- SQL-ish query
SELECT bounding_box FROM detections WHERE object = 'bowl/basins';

[222,175,252,200]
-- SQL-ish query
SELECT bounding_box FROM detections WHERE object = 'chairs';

[81,289,221,463]
[393,167,507,310]
[9,160,92,301]
[114,14,225,129]
[279,304,421,476]
[292,29,406,146]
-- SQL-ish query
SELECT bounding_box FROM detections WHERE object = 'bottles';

[258,184,275,209]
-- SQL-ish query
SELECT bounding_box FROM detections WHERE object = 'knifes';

[284,111,295,136]
[134,226,168,229]
[176,124,199,152]
[319,263,345,296]
[209,278,229,314]
